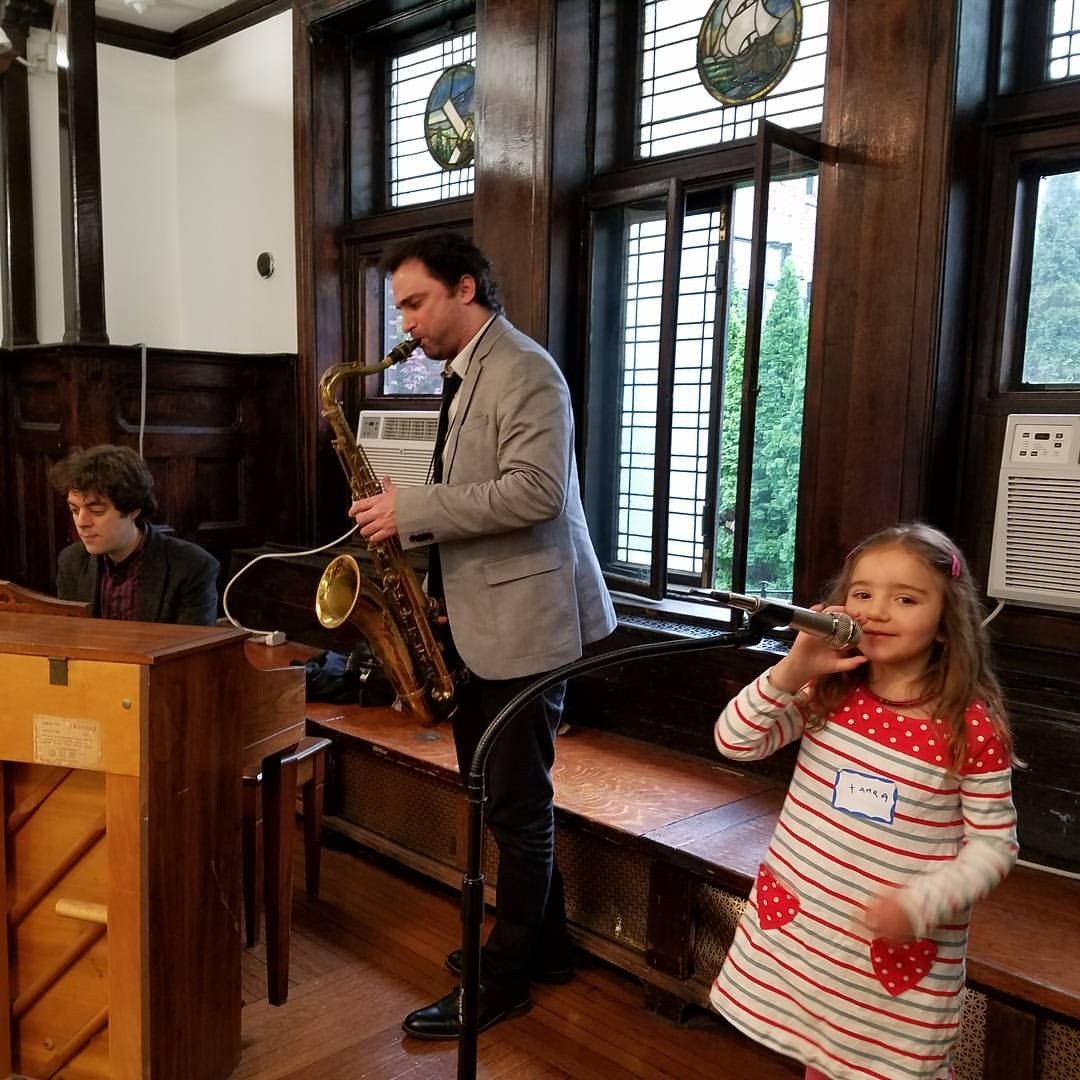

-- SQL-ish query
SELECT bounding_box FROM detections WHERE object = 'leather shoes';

[401,975,533,1040]
[445,943,578,986]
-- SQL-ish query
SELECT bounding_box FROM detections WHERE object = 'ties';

[426,370,463,597]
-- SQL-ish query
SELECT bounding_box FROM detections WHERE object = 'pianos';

[0,577,307,1080]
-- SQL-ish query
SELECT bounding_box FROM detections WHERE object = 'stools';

[243,737,332,947]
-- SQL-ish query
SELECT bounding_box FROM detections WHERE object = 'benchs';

[306,701,1080,1080]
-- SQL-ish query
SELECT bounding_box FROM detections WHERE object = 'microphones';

[711,590,862,653]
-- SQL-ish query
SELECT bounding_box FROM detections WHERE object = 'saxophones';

[314,333,463,732]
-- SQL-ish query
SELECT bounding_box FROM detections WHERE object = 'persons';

[338,229,619,1041]
[48,444,219,629]
[712,523,1020,1080]
